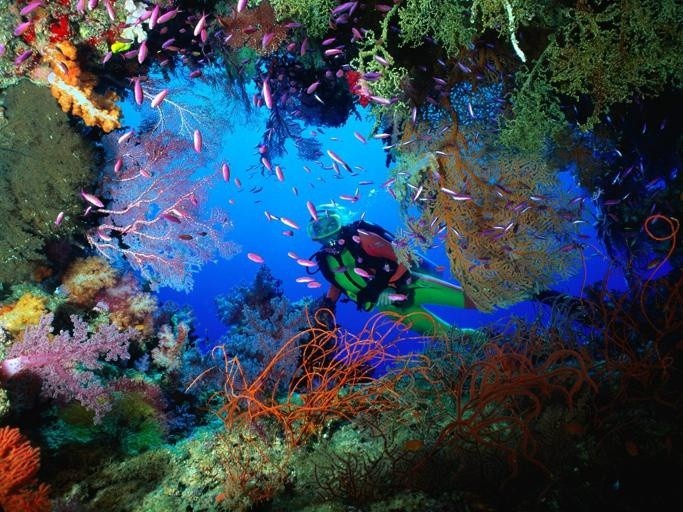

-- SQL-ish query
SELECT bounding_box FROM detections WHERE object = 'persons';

[305,204,487,348]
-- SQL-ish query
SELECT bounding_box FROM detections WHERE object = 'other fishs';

[1,1,683,306]
